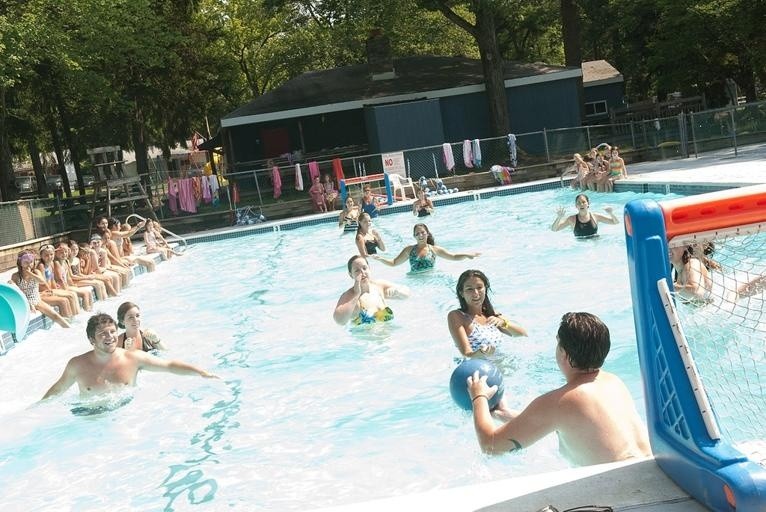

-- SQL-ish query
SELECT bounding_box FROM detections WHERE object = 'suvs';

[47,174,63,191]
[17,175,37,194]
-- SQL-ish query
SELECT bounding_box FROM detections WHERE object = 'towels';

[164,174,225,214]
[442,133,517,169]
[271,158,344,199]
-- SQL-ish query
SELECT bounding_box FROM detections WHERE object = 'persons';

[466,312,652,469]
[354,212,386,258]
[309,174,338,213]
[552,194,619,237]
[572,146,626,194]
[38,313,221,418]
[331,254,409,327]
[10,217,175,331]
[110,301,164,359]
[667,246,711,302]
[366,223,482,273]
[446,269,529,360]
[413,189,434,217]
[358,184,380,218]
[339,197,364,233]
[691,240,722,271]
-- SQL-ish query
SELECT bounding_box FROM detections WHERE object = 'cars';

[73,175,96,189]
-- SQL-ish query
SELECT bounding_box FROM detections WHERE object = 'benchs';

[334,189,361,209]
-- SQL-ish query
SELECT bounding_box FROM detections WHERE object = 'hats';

[89,233,101,241]
[107,217,119,229]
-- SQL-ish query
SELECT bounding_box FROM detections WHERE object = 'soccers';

[237,212,264,224]
[420,176,458,197]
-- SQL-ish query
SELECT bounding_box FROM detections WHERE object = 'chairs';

[388,173,417,202]
[86,144,164,243]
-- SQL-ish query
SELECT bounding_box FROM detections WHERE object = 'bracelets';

[470,394,488,404]
[502,319,509,330]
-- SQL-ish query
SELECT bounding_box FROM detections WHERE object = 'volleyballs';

[449,360,503,411]
